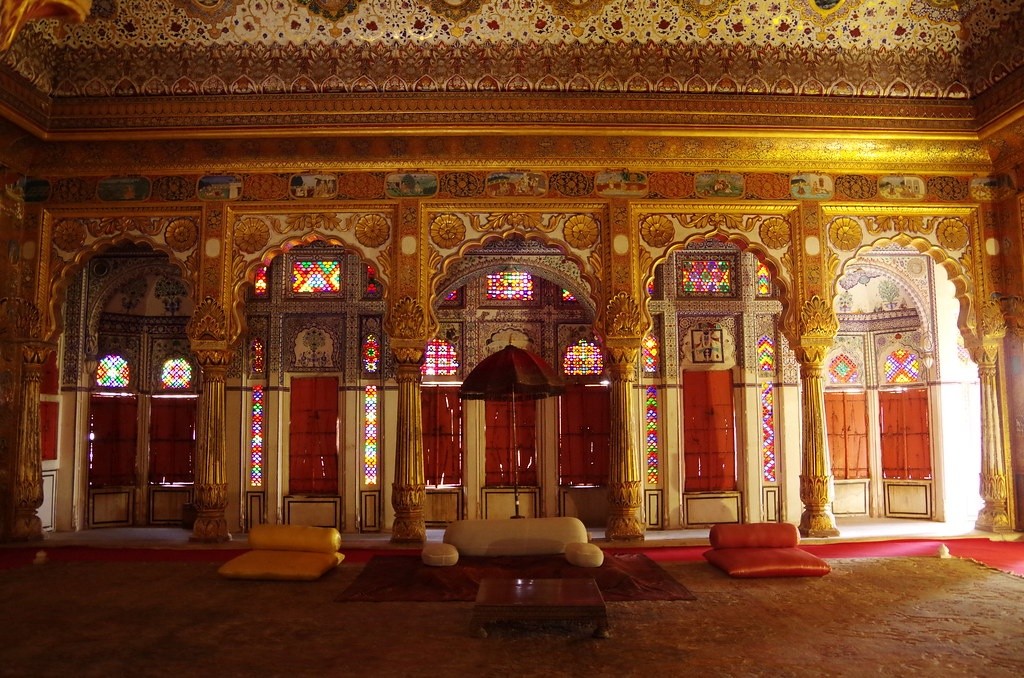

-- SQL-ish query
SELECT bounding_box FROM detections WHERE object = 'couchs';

[411,517,627,589]
[215,523,345,583]
[702,522,832,577]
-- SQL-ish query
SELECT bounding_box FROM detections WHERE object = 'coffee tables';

[470,577,610,641]
